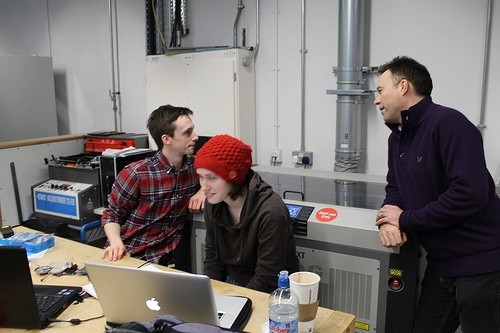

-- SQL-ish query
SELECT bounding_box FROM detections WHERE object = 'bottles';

[269,271,299,333]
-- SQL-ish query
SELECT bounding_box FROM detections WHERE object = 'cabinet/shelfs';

[145,48,256,164]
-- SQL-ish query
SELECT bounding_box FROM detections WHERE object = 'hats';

[194,134,253,185]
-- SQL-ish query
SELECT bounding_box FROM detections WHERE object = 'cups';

[289,271,321,333]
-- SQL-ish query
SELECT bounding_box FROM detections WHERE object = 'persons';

[194,134,303,296]
[99,104,208,274]
[374,55,500,332]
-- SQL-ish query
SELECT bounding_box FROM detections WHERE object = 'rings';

[380,213,384,217]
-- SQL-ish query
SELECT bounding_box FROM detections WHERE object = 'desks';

[0,225,357,333]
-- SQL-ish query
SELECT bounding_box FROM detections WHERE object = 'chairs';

[81,220,105,244]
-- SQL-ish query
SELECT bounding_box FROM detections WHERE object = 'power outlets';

[270,149,282,163]
[292,151,313,165]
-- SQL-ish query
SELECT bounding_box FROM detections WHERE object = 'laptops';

[0,246,82,330]
[84,262,252,331]
